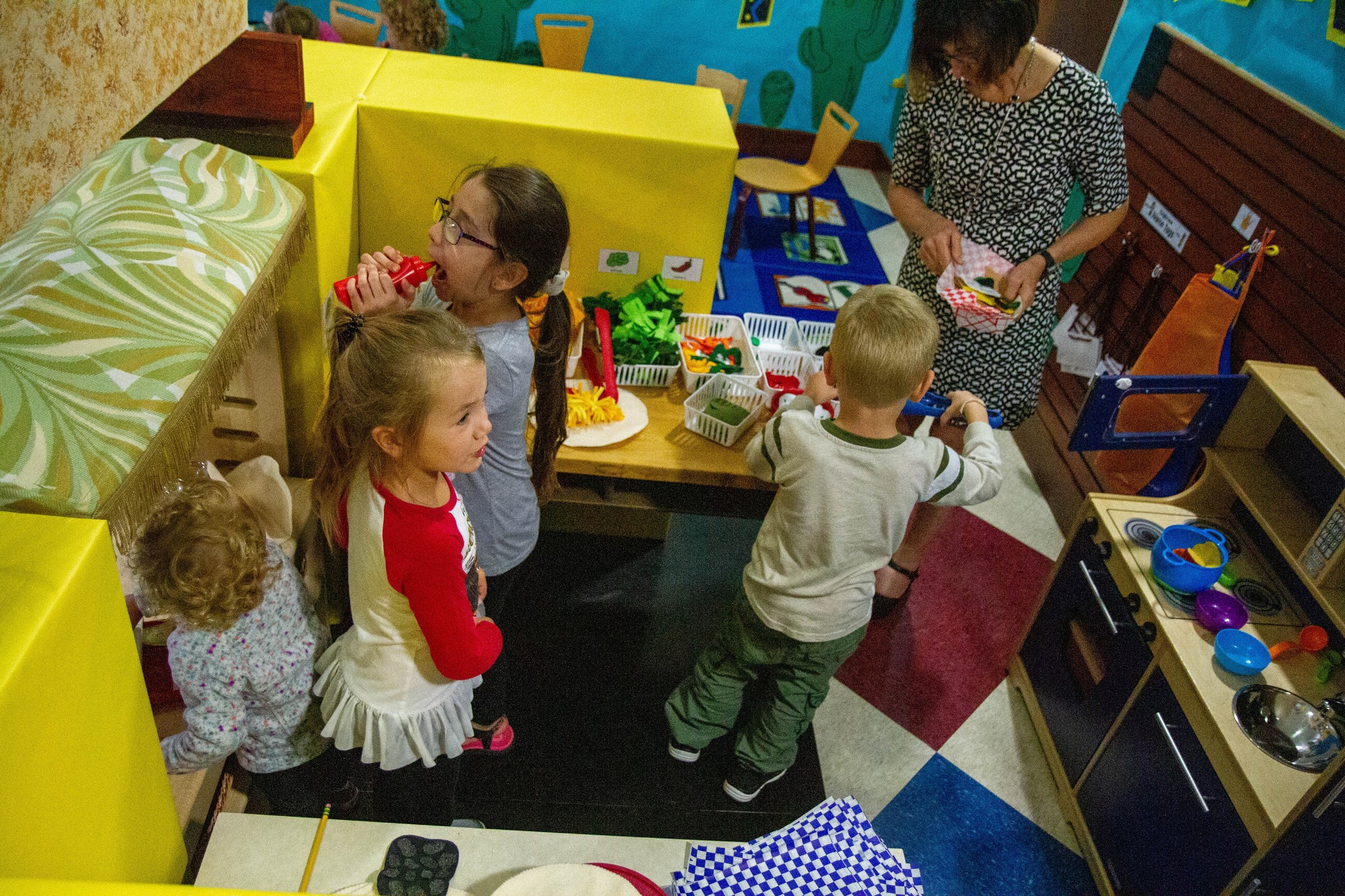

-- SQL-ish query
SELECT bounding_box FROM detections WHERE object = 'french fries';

[563,381,625,428]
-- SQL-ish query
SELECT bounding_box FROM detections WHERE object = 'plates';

[526,378,649,448]
[490,863,669,896]
[330,882,475,896]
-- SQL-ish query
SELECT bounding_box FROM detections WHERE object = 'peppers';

[680,336,732,372]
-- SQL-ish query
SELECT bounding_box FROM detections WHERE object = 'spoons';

[1267,625,1328,661]
[1315,650,1343,683]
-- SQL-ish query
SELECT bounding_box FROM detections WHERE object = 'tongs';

[900,391,1003,428]
[581,307,618,405]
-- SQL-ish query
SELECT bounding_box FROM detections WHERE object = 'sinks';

[1232,685,1342,775]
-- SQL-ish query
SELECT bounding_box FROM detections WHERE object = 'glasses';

[432,196,519,264]
[928,47,985,66]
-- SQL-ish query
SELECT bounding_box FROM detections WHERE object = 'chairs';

[726,98,860,264]
[328,0,383,46]
[695,64,748,132]
[533,14,594,73]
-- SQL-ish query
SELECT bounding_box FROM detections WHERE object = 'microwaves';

[1068,359,1345,589]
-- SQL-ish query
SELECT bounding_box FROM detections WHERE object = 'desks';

[513,329,777,492]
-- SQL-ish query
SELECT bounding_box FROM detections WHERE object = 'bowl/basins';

[1214,628,1271,676]
[1232,683,1343,774]
[1196,590,1249,635]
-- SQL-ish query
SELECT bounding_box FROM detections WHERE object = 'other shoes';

[724,767,787,802]
[314,780,359,809]
[450,818,486,829]
[460,717,514,750]
[870,559,922,617]
[668,734,701,762]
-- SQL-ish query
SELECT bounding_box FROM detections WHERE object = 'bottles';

[333,256,437,311]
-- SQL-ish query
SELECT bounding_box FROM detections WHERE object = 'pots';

[1151,524,1229,595]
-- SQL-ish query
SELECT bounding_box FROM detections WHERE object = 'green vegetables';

[689,344,744,375]
[581,275,688,385]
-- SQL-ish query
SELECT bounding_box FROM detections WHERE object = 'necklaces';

[933,30,1040,234]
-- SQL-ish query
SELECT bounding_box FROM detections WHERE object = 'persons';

[664,282,1002,804]
[124,477,363,821]
[869,0,1132,619]
[377,0,447,57]
[269,0,344,45]
[348,159,578,750]
[306,307,505,778]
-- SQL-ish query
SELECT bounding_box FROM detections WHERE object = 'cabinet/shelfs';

[1007,360,1345,896]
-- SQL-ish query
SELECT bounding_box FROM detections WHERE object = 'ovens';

[1012,522,1152,788]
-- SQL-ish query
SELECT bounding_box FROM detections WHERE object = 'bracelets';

[959,397,987,422]
[1034,249,1059,282]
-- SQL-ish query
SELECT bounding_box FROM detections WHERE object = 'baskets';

[674,312,762,394]
[612,360,682,387]
[816,359,840,422]
[683,372,770,448]
[798,321,836,359]
[757,349,819,408]
[742,312,806,354]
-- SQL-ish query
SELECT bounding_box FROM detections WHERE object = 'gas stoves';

[1107,507,1305,627]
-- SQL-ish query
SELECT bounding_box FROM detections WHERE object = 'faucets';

[1318,692,1345,723]
